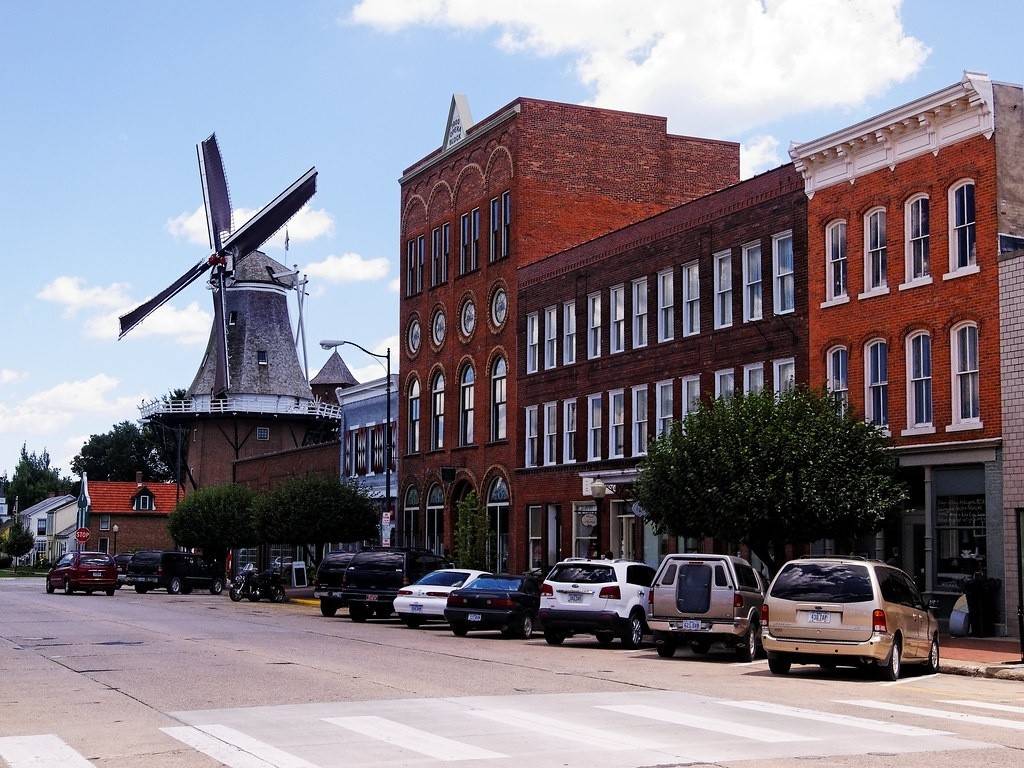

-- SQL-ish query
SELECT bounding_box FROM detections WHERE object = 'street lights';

[320,340,392,547]
[137,419,181,551]
[592,474,606,560]
[113,525,119,556]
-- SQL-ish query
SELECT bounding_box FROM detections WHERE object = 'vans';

[648,553,768,662]
[760,554,939,682]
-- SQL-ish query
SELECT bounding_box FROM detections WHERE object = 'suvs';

[341,547,454,622]
[538,558,657,649]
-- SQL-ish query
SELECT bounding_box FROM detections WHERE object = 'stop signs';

[75,527,91,542]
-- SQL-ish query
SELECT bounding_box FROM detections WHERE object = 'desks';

[921,591,963,608]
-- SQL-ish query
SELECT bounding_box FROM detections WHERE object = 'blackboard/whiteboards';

[292,567,307,588]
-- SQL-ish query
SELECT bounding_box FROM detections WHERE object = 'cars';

[46,552,118,596]
[313,550,358,617]
[273,557,293,570]
[444,574,543,639]
[392,569,494,627]
[937,557,980,589]
[112,552,134,590]
[124,549,227,595]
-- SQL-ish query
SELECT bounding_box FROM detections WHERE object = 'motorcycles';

[229,563,286,603]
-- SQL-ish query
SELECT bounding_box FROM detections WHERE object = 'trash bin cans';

[960,579,1002,637]
